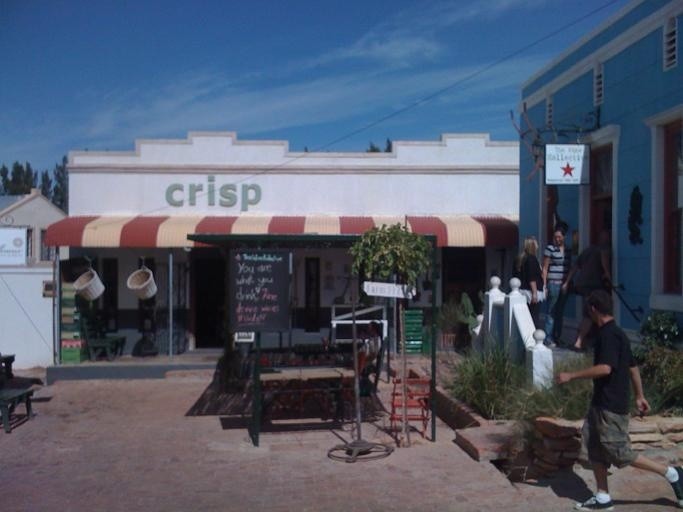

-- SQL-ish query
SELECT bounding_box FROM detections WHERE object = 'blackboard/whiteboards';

[224,247,292,332]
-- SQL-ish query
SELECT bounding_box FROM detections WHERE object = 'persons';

[516,234,542,331]
[560,232,610,352]
[541,227,570,350]
[553,287,682,512]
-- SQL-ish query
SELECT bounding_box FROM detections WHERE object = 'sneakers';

[573,494,614,512]
[668,465,682,507]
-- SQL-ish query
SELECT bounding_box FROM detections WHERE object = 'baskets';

[72,269,108,303]
[126,267,160,302]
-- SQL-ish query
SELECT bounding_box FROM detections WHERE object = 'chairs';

[82,316,123,361]
[0,354,34,434]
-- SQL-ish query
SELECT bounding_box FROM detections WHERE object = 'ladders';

[390,376,432,438]
